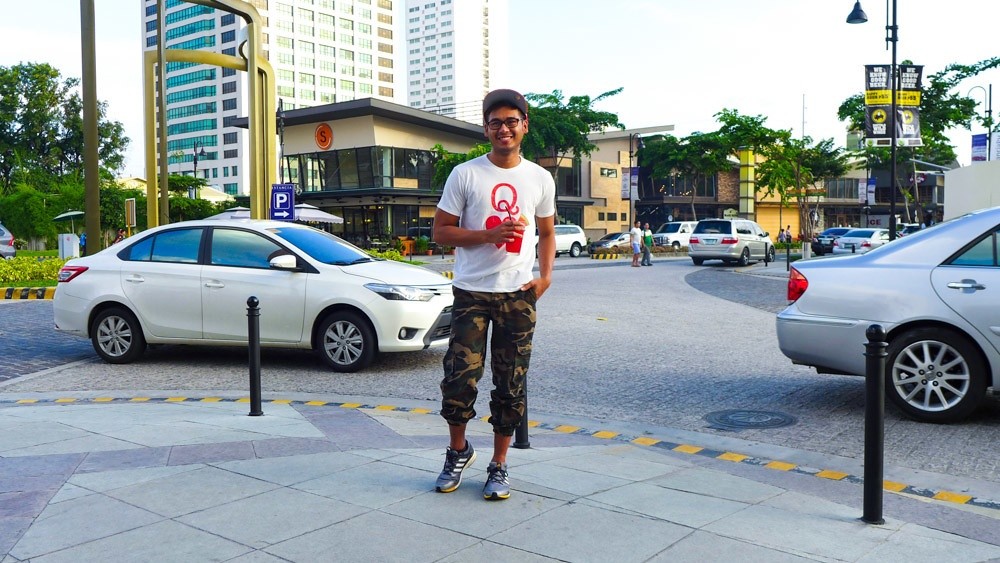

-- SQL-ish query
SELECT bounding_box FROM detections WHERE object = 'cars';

[587,232,631,254]
[833,228,904,255]
[777,205,1000,424]
[901,223,927,238]
[0,220,17,259]
[810,227,854,256]
[54,217,455,374]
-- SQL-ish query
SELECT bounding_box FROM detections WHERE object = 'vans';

[535,225,587,258]
[406,226,431,240]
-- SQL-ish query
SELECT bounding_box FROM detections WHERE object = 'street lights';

[629,133,647,231]
[193,140,207,199]
[275,98,287,184]
[961,83,992,162]
[847,0,898,243]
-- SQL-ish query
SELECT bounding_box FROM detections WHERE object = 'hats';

[483,88,529,122]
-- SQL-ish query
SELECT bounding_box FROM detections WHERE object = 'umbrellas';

[293,203,344,225]
[202,205,250,220]
[52,208,85,233]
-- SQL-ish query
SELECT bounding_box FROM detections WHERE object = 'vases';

[448,250,455,255]
[425,250,433,255]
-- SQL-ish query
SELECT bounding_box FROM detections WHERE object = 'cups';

[506,221,525,254]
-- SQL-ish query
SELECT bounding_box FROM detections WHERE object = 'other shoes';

[632,264,641,267]
[641,263,648,266]
[647,264,653,266]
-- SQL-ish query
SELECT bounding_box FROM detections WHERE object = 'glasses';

[486,118,524,130]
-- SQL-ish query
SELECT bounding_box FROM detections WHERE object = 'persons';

[431,88,557,504]
[80,230,87,258]
[641,222,656,266]
[629,220,643,267]
[110,228,127,246]
[779,228,786,243]
[786,225,792,243]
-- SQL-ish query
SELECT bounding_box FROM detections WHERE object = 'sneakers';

[482,461,511,500]
[435,439,478,493]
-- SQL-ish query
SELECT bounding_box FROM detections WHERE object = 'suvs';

[652,221,699,251]
[687,218,776,267]
